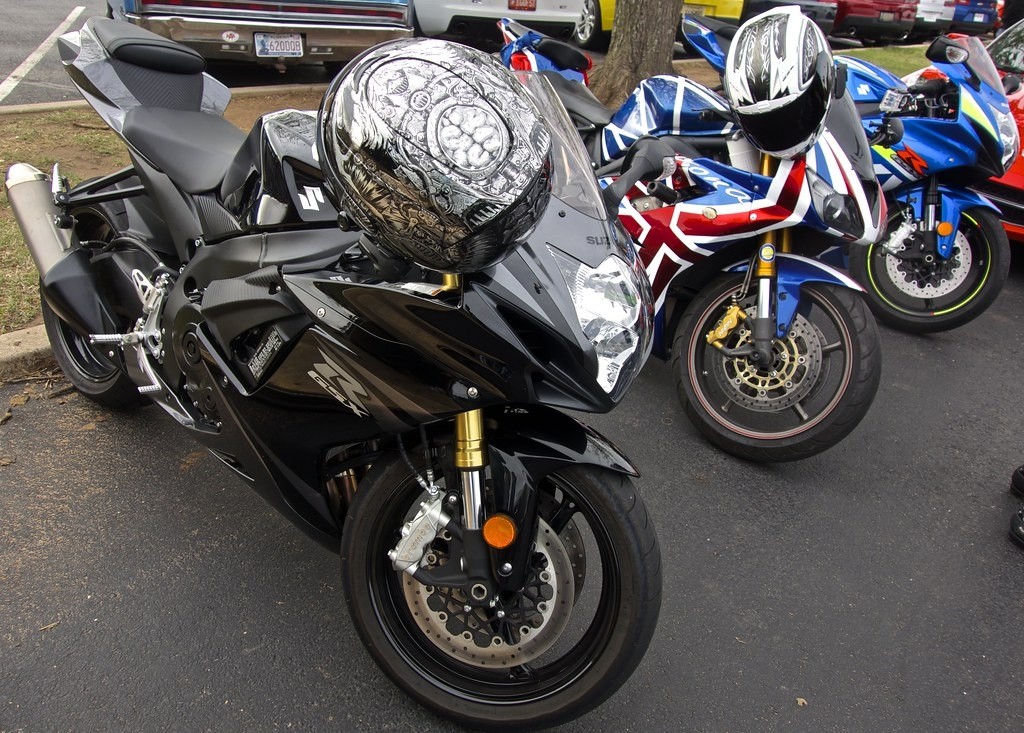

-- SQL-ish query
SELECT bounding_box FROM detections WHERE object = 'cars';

[967,17,1024,244]
[410,0,584,42]
[738,0,1006,48]
[573,0,745,56]
[105,0,416,70]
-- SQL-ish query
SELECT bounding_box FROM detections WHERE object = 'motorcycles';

[491,17,890,461]
[2,13,665,733]
[679,12,1021,335]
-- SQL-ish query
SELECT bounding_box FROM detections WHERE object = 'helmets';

[317,37,555,274]
[724,5,837,159]
